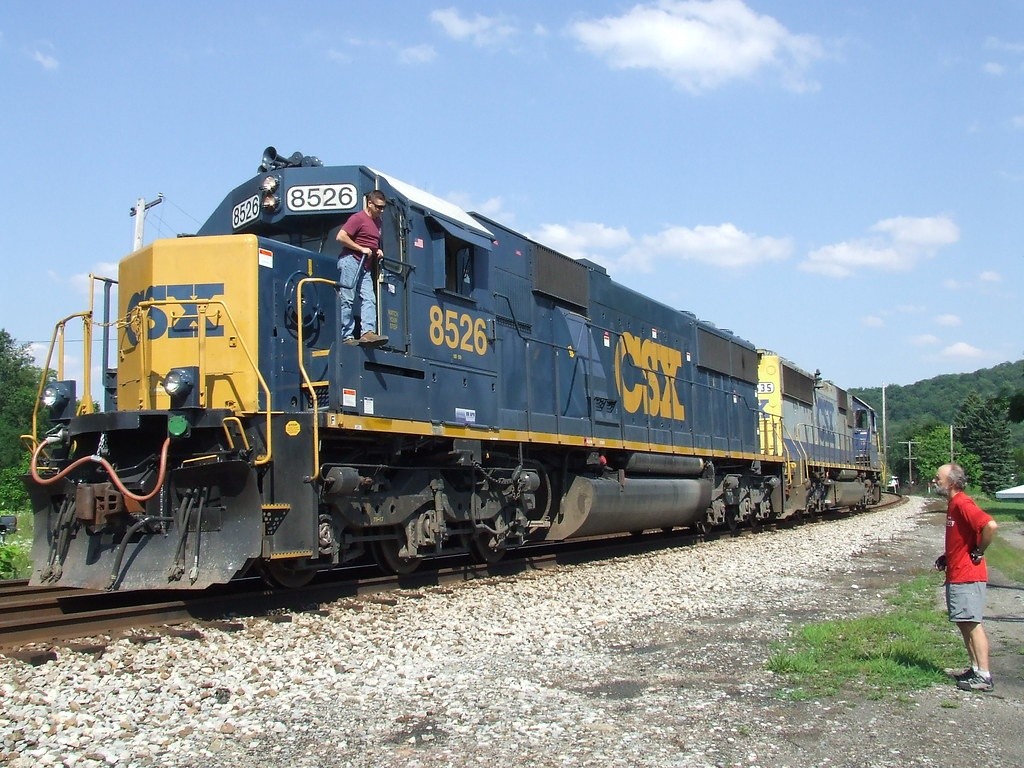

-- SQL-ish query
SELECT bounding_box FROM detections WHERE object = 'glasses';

[371,200,386,210]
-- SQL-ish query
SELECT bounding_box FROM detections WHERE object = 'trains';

[18,147,884,593]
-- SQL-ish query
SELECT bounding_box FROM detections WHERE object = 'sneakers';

[950,667,976,681]
[359,330,389,348]
[343,338,359,346]
[956,671,994,692]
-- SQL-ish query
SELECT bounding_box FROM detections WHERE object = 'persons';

[336,190,388,346]
[932,463,997,691]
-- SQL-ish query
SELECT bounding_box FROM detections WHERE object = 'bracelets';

[975,546,984,554]
